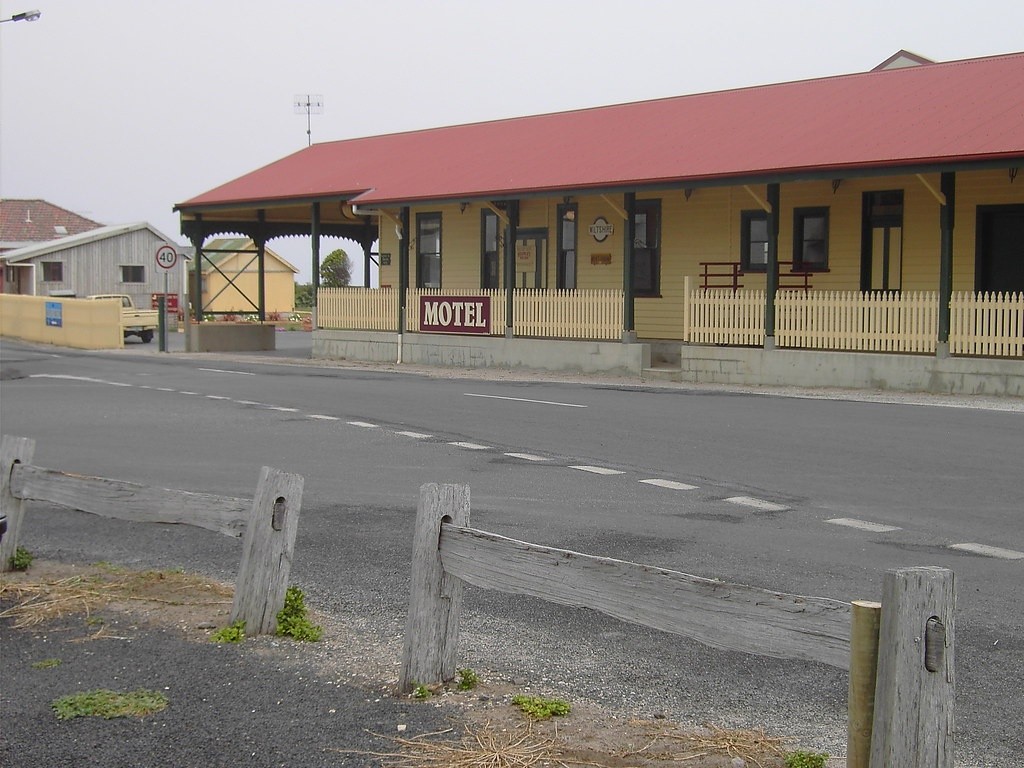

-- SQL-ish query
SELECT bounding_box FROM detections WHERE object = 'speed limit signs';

[155,241,178,273]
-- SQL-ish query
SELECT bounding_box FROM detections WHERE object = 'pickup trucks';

[86,293,160,343]
